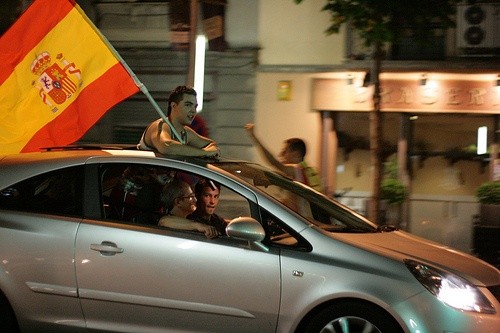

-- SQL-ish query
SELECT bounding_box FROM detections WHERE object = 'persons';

[244,123,332,226]
[158,182,221,235]
[187,180,227,235]
[109,86,223,224]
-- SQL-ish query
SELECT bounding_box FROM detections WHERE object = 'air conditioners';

[456,3,500,48]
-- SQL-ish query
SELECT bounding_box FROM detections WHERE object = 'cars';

[1,146,494,333]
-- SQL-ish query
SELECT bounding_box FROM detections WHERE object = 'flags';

[2,0,139,154]
